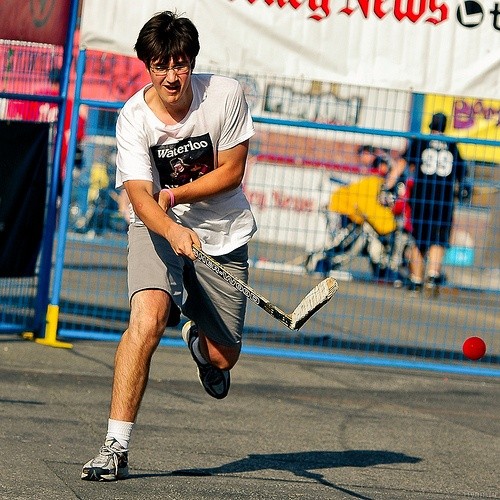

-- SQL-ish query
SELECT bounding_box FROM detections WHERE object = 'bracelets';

[162,188,175,208]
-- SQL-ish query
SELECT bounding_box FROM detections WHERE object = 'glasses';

[150,57,194,73]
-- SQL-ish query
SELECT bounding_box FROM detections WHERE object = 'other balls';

[463,336,486,360]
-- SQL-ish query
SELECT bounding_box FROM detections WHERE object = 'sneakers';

[182,320,231,400]
[81,437,129,481]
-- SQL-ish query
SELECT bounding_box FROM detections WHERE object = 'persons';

[379,113,470,297]
[80,12,257,481]
[305,145,412,289]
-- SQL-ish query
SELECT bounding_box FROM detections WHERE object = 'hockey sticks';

[188,241,339,332]
